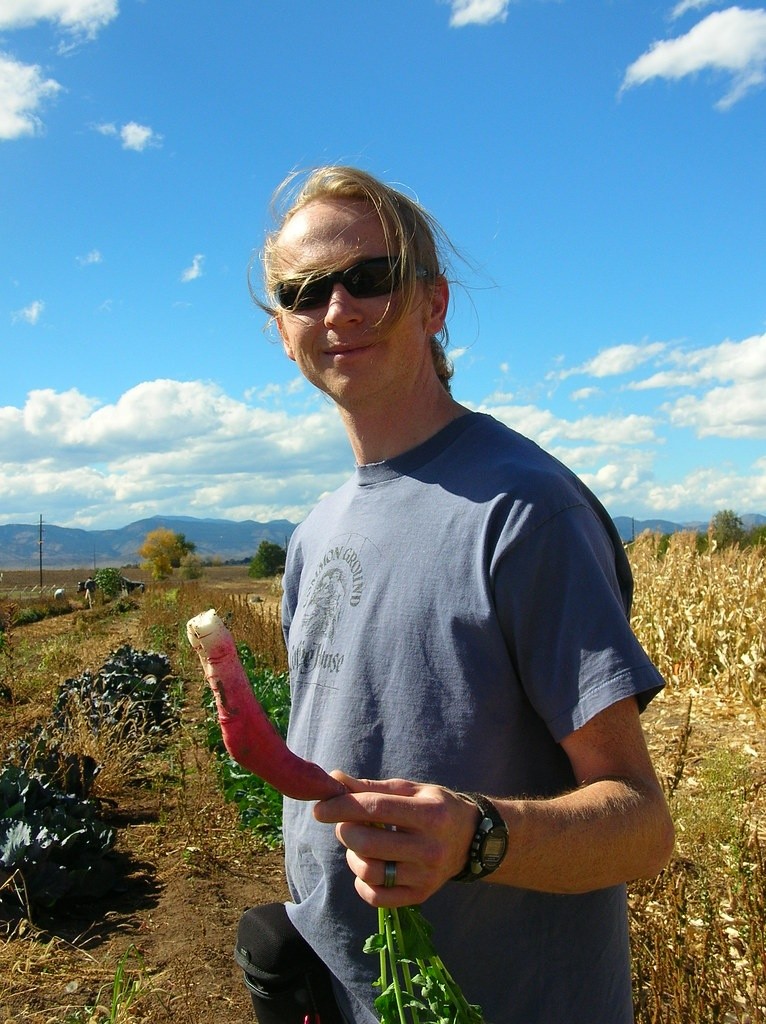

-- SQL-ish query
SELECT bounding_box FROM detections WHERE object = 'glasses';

[272,256,434,311]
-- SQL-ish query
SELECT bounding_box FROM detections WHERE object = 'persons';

[262,165,675,1024]
[84,576,96,609]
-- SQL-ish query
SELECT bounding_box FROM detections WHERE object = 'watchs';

[452,791,510,885]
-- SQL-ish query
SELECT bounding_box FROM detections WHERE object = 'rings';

[384,862,397,888]
